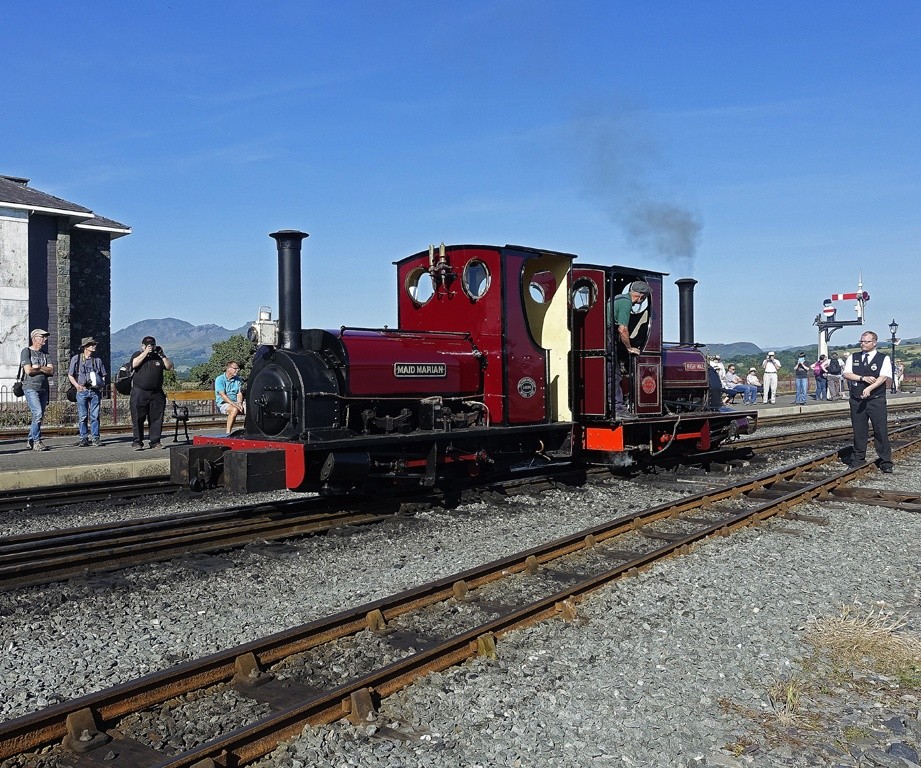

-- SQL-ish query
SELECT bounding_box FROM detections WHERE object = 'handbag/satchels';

[67,386,78,403]
[13,382,24,397]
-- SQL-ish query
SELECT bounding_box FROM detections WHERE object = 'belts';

[766,372,777,374]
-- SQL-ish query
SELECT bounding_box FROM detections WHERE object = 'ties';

[863,354,869,365]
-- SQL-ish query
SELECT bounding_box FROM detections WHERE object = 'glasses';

[732,369,735,370]
[859,340,872,345]
[35,335,47,338]
[230,366,241,371]
[751,371,756,372]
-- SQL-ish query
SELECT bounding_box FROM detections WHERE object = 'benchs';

[166,391,247,445]
[723,378,770,404]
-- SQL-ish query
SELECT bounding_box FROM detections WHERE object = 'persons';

[762,352,781,405]
[710,355,771,405]
[895,359,904,390]
[215,361,246,433]
[811,351,851,401]
[793,352,810,405]
[129,336,174,451]
[68,337,107,447]
[607,281,652,418]
[843,331,894,473]
[20,329,54,451]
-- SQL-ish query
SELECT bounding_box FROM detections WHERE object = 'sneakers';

[149,442,166,449]
[26,440,33,449]
[33,441,50,451]
[79,438,90,447]
[92,438,106,447]
[131,442,144,450]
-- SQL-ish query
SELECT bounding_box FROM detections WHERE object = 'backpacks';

[115,361,135,395]
[828,358,841,374]
[813,362,823,376]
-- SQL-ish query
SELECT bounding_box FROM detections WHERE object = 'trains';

[169,227,759,504]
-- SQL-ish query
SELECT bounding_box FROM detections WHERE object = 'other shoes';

[884,467,893,473]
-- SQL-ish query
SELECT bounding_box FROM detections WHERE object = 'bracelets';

[860,376,864,381]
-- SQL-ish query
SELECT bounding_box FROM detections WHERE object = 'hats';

[78,336,98,350]
[768,352,775,355]
[31,329,50,339]
[142,336,156,345]
[748,367,757,373]
[632,281,654,293]
[798,352,806,357]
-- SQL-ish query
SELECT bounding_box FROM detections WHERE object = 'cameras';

[150,345,162,354]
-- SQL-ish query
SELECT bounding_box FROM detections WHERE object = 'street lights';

[887,319,900,395]
